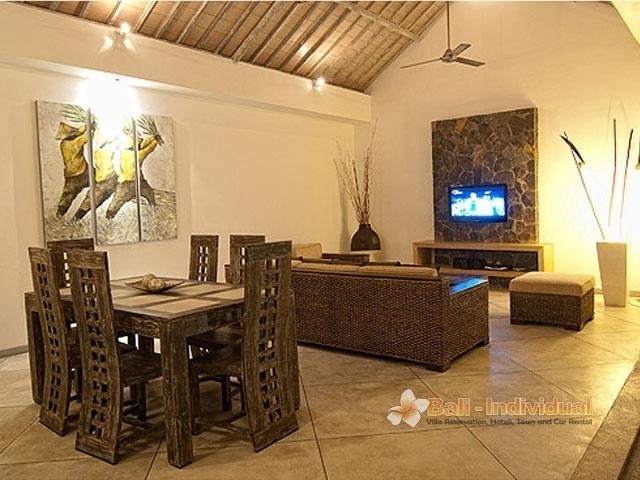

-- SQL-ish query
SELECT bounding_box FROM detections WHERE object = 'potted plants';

[332,116,381,251]
[558,120,633,307]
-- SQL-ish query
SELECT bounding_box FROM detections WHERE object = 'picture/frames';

[35,100,95,242]
[134,116,178,242]
[89,108,140,247]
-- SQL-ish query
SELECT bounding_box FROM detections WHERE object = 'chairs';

[187,234,266,412]
[187,240,299,453]
[45,239,95,288]
[190,236,218,284]
[29,248,135,437]
[66,250,164,465]
[295,242,368,263]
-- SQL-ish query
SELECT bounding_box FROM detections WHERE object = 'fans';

[401,1,485,71]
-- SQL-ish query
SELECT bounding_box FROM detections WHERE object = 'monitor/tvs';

[449,183,507,223]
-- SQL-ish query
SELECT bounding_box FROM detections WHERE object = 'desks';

[26,277,301,468]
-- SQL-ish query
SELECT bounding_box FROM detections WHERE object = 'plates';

[126,278,183,295]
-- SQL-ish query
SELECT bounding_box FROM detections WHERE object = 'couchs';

[224,257,490,373]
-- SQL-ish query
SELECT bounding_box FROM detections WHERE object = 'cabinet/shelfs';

[413,240,545,281]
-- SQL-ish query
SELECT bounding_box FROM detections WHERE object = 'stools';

[509,269,595,330]
